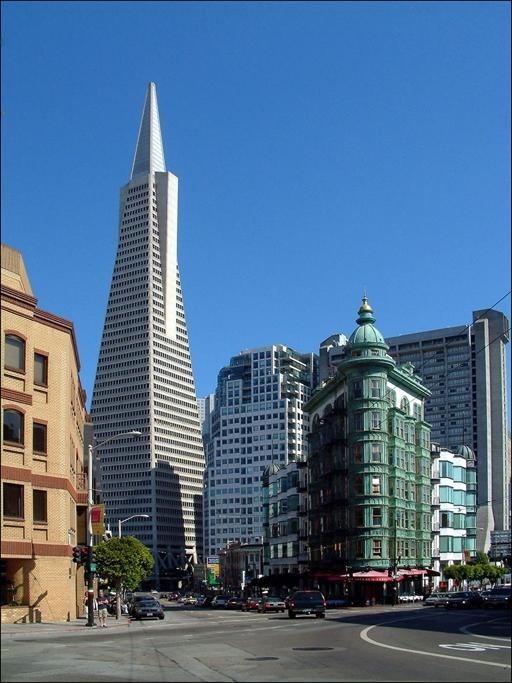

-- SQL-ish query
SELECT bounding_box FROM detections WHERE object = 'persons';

[97,590,110,628]
[83,591,89,619]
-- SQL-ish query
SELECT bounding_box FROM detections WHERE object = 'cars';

[126,591,286,620]
[425,588,512,611]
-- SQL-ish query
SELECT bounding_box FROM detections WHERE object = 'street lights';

[86,430,142,626]
[116,514,149,620]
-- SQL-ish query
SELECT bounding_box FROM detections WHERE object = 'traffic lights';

[72,545,92,563]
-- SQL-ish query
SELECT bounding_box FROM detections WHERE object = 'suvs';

[287,590,326,619]
